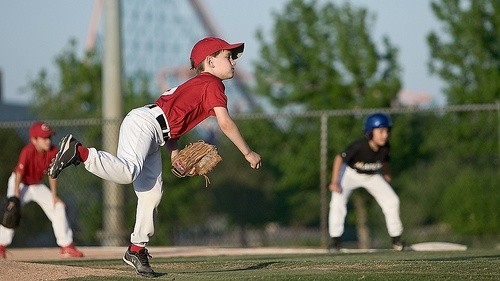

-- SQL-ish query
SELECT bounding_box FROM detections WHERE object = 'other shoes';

[328,241,339,253]
[392,242,411,250]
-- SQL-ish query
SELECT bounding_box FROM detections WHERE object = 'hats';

[190,38,244,69]
[29,123,57,137]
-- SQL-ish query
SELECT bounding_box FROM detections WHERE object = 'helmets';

[364,114,392,139]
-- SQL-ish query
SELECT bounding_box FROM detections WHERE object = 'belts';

[149,105,169,142]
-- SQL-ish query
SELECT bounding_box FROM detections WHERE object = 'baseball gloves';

[171,140,224,178]
[0,196,22,228]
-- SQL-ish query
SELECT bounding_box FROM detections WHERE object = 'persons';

[327,113,404,251]
[0,121,83,260]
[47,37,262,278]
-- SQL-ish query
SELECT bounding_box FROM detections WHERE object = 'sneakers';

[0,245,6,259]
[48,133,82,179]
[61,244,83,257]
[122,245,154,277]
